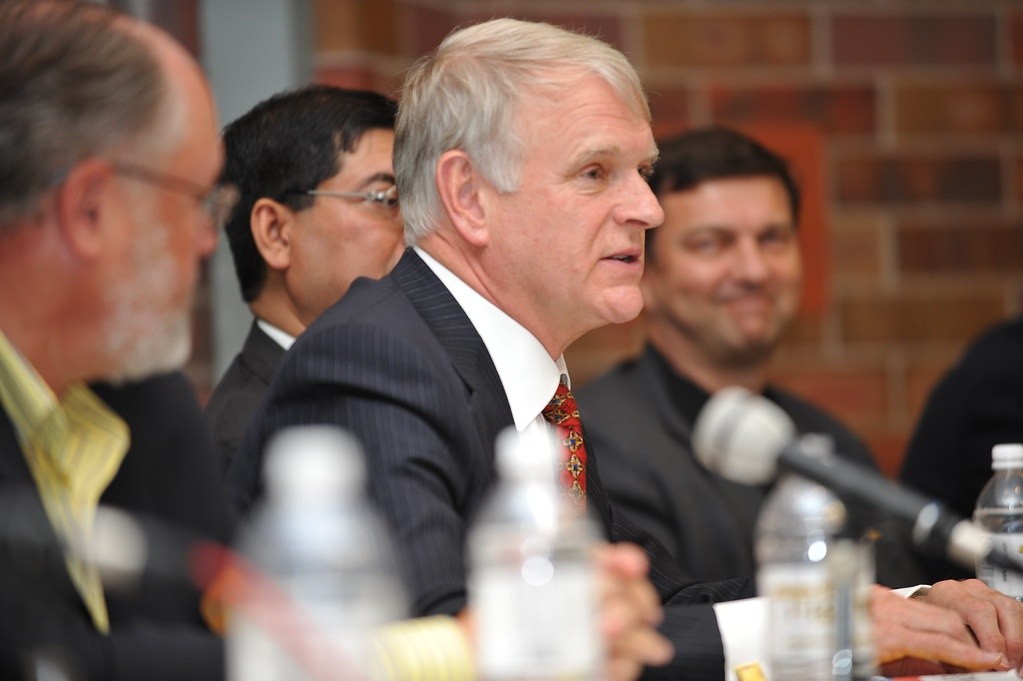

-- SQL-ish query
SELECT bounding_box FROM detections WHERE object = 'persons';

[0,1,239,681]
[202,1,1023,681]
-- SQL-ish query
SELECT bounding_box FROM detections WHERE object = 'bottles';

[973,443,1023,603]
[753,433,857,681]
[223,422,403,681]
[465,424,605,681]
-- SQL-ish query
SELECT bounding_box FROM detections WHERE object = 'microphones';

[692,387,995,565]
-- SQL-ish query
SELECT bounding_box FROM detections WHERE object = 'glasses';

[114,162,238,238]
[307,184,397,216]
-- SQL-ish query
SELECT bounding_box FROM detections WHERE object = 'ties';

[541,374,587,523]
